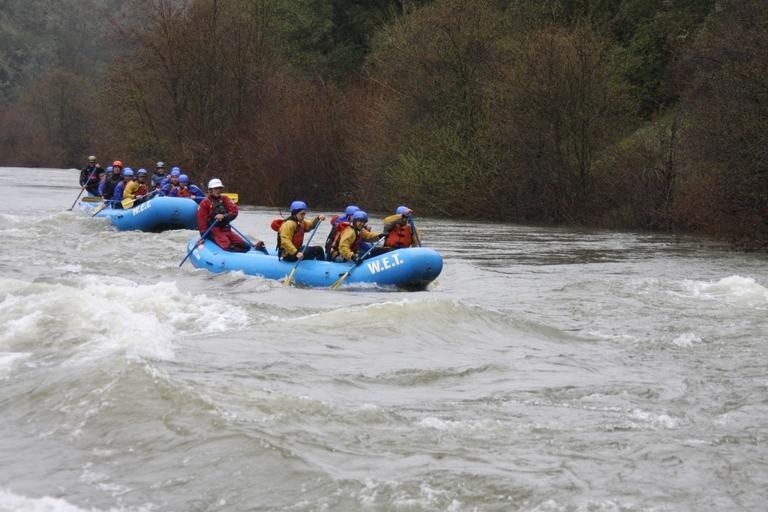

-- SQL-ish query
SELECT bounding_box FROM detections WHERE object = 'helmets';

[88,155,96,163]
[170,167,188,183]
[155,161,164,168]
[207,178,223,190]
[353,212,368,223]
[290,201,307,213]
[136,169,147,176]
[346,206,360,216]
[396,206,408,215]
[106,160,133,176]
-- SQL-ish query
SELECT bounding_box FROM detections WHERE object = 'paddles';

[282,216,324,286]
[82,195,121,204]
[330,237,384,290]
[195,192,239,204]
[121,190,158,209]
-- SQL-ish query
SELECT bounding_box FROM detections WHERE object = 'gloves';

[352,257,363,266]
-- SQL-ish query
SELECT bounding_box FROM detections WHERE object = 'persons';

[326,206,362,259]
[196,178,250,252]
[384,206,418,251]
[276,200,325,261]
[331,212,385,265]
[80,156,203,209]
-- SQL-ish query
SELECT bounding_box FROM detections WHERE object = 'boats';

[79,189,201,232]
[186,234,445,289]
